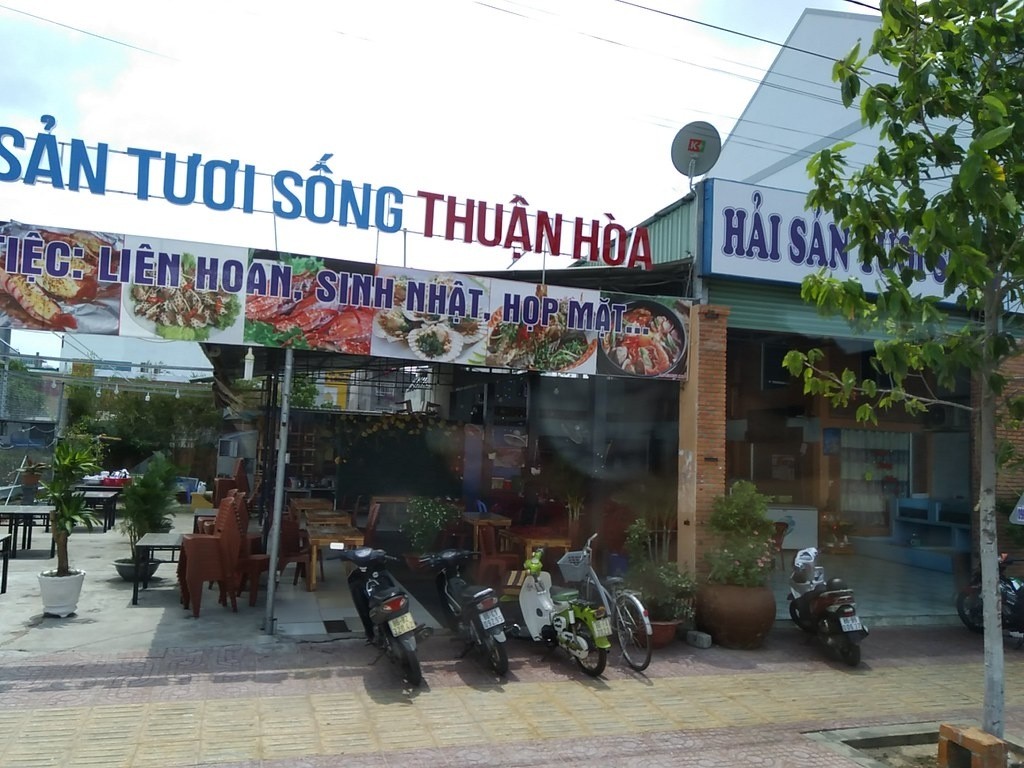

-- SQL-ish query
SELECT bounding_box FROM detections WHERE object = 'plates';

[491,301,598,371]
[122,273,234,337]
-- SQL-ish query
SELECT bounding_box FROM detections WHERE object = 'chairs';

[360,501,379,560]
[175,457,324,620]
[479,526,520,591]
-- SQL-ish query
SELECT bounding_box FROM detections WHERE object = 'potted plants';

[35,433,101,618]
[17,455,53,492]
[625,521,683,647]
[111,452,179,580]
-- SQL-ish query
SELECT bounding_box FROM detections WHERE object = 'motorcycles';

[417,548,521,675]
[955,552,1024,635]
[497,545,614,676]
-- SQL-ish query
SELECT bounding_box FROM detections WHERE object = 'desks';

[0,505,62,558]
[457,509,512,553]
[290,499,364,592]
[497,526,572,567]
[48,492,119,532]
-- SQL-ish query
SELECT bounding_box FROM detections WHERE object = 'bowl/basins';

[81,477,103,485]
[601,299,687,377]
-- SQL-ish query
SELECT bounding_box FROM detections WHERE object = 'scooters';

[787,546,870,667]
[330,542,434,687]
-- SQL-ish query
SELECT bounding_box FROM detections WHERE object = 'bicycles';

[558,533,653,672]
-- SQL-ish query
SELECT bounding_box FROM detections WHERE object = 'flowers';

[708,481,779,585]
[398,496,461,554]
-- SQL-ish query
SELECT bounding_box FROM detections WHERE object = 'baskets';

[557,550,590,583]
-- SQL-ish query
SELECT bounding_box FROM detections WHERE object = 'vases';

[405,552,434,574]
[697,581,775,649]
